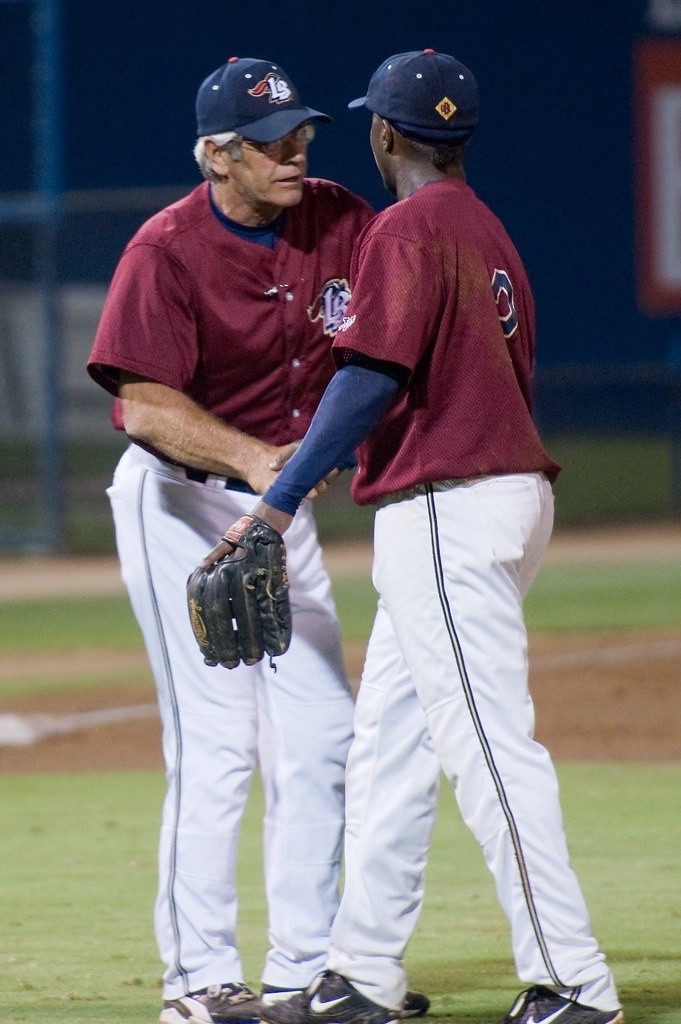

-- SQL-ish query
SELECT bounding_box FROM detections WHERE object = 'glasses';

[226,125,315,153]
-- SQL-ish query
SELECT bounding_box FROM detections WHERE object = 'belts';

[184,466,259,496]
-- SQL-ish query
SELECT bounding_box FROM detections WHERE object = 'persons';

[85,54,377,1024]
[184,47,627,1024]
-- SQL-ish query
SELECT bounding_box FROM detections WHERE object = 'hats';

[348,49,480,139]
[195,57,333,142]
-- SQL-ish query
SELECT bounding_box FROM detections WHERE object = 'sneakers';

[159,982,264,1024]
[262,985,430,1021]
[261,971,403,1024]
[496,984,624,1024]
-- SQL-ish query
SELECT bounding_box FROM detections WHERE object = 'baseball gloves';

[184,511,295,671]
